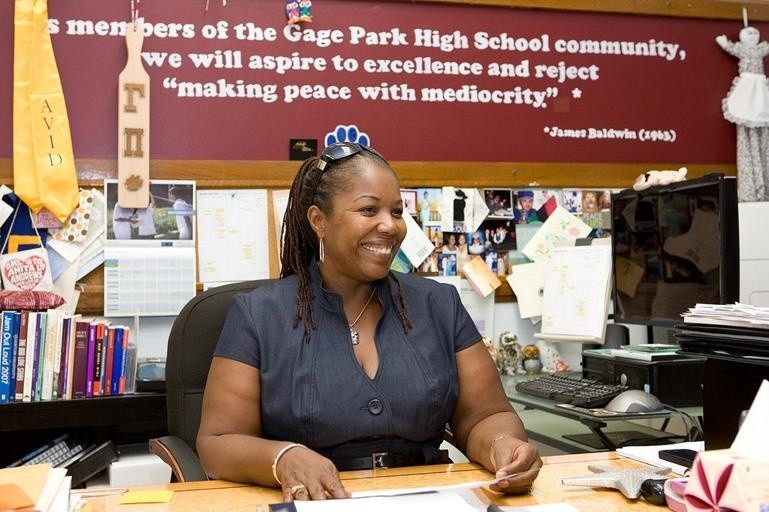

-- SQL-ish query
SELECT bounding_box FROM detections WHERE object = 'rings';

[291,484,307,497]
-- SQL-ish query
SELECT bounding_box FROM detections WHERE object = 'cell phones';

[659,449,698,468]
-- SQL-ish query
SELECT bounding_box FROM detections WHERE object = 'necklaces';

[348,286,377,345]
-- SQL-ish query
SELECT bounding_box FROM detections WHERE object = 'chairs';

[149,275,458,481]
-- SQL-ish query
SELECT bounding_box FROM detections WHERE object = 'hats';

[518,191,534,200]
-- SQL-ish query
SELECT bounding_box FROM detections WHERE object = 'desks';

[68,446,757,512]
[1,390,166,432]
[675,350,769,447]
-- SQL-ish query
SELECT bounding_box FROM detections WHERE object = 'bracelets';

[489,433,513,469]
[271,442,308,486]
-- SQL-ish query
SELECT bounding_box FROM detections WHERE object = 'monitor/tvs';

[610,178,739,327]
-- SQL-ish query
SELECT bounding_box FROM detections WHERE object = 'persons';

[422,190,540,276]
[167,188,192,240]
[130,192,157,239]
[196,142,543,505]
[112,202,136,239]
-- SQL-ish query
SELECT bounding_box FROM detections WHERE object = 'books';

[0,309,139,405]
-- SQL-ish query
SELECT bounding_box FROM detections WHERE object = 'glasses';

[308,142,390,208]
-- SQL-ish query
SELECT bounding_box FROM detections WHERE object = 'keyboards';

[516,373,623,410]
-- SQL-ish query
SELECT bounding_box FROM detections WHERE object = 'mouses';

[605,387,662,413]
